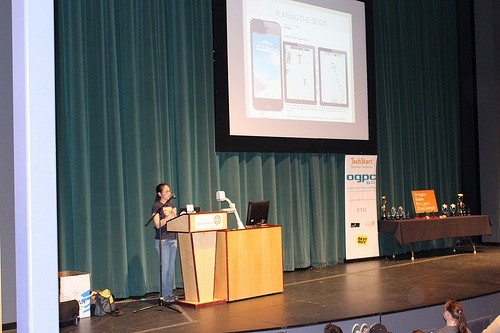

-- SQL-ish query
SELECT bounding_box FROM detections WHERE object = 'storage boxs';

[60,271,92,319]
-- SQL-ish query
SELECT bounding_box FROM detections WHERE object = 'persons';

[434,300,473,333]
[151,183,178,303]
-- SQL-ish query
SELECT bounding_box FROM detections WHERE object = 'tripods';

[133,197,182,314]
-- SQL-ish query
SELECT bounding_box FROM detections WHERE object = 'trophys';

[381,196,410,220]
[441,194,471,216]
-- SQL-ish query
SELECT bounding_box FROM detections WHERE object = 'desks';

[374,215,494,263]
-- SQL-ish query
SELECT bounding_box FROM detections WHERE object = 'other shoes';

[352,323,360,333]
[360,323,368,333]
[160,295,178,304]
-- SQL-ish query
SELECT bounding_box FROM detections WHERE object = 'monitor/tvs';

[246,201,269,228]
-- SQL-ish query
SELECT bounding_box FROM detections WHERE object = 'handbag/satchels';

[95,291,116,317]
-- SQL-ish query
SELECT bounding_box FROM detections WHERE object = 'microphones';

[172,194,176,199]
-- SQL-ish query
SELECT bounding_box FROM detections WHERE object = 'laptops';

[180,207,200,213]
